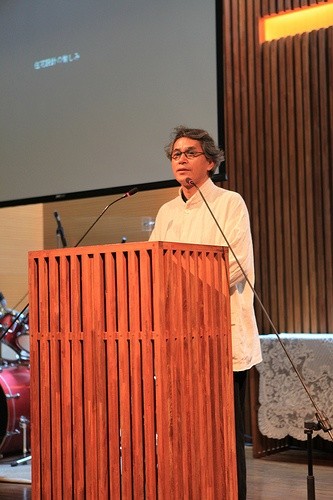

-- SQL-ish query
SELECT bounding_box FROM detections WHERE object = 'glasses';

[171,150,205,159]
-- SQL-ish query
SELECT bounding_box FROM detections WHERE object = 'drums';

[16,332,30,364]
[0,361,31,459]
[0,309,25,346]
[0,340,20,369]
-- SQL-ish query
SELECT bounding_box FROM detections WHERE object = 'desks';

[247,333,333,457]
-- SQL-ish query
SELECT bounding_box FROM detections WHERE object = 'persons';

[149,125,262,500]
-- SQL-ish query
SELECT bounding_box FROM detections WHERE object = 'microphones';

[54,211,67,247]
[0,188,138,339]
[185,177,333,440]
